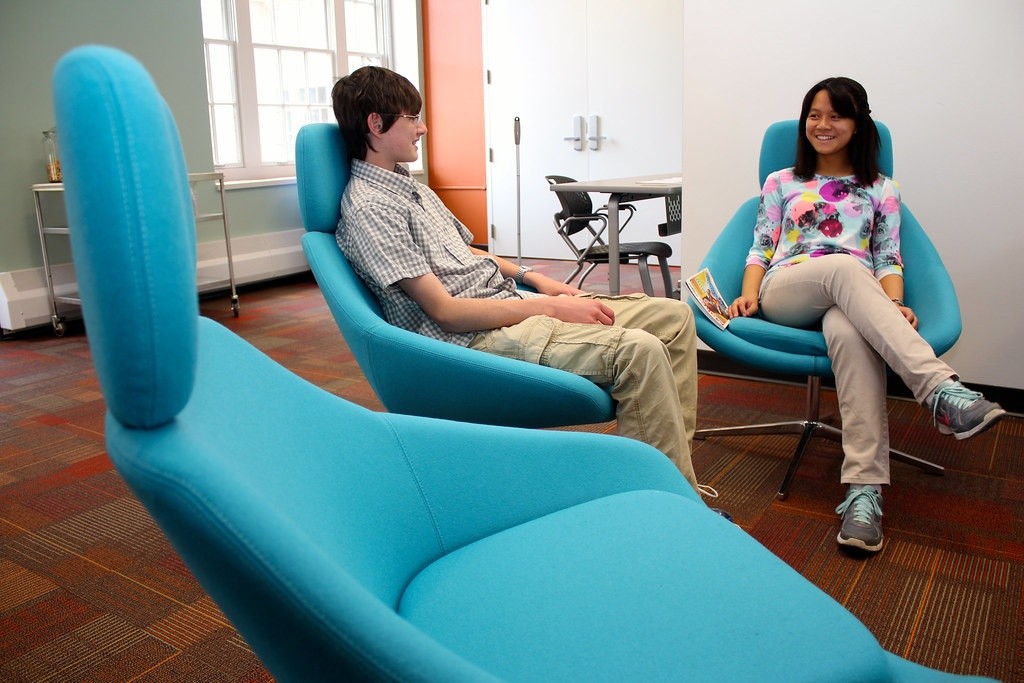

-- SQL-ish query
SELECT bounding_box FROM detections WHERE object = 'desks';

[550,172,683,297]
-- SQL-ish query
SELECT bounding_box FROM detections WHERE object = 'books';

[684,268,731,330]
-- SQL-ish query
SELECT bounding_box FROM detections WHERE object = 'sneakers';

[836,485,884,551]
[926,381,1006,440]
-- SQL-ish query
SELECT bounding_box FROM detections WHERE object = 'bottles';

[42,129,65,184]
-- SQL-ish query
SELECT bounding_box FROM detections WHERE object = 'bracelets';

[891,297,904,306]
[517,265,532,283]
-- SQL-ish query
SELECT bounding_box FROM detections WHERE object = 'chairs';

[294,123,622,430]
[544,175,675,300]
[53,43,1006,683]
[683,116,964,501]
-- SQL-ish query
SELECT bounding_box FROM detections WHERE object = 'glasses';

[379,112,421,126]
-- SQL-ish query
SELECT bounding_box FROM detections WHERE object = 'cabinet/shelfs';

[32,172,243,337]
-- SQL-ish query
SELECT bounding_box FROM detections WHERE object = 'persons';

[332,67,733,523]
[725,77,1006,551]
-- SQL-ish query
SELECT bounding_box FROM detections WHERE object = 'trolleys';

[31,172,241,338]
